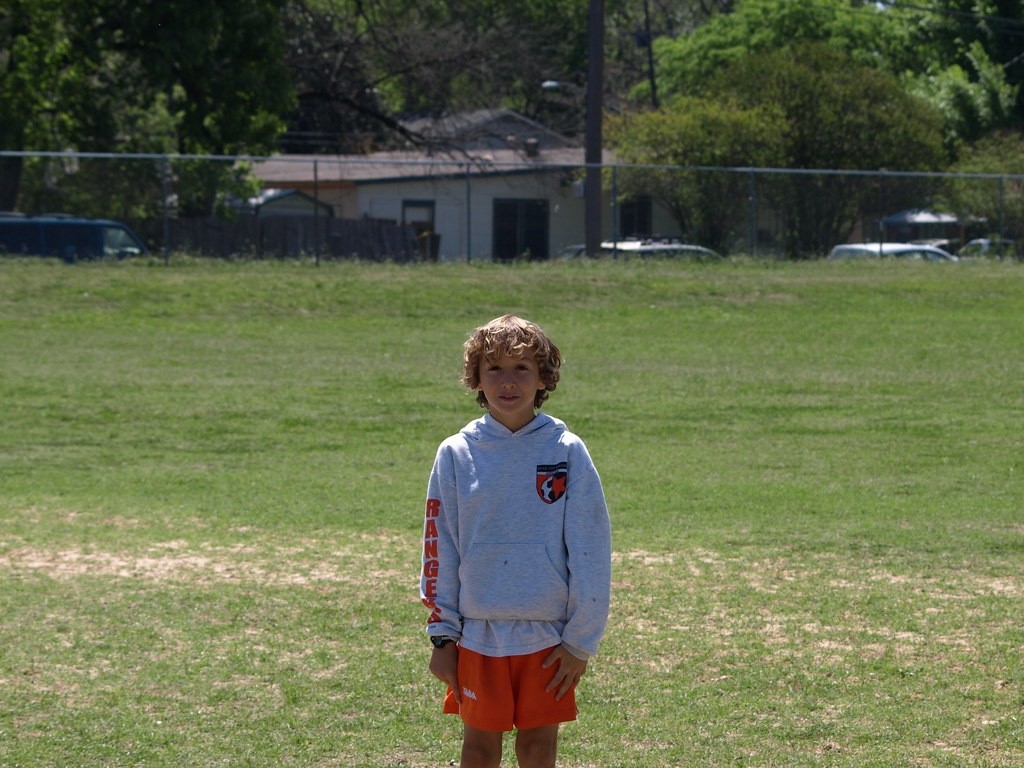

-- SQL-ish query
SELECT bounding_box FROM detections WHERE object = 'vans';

[0,209,154,260]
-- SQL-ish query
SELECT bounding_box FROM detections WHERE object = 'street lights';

[542,1,603,257]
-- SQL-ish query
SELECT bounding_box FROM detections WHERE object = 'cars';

[826,242,959,264]
[958,237,1019,263]
[552,235,722,263]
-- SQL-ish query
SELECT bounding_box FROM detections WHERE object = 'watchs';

[429,634,454,648]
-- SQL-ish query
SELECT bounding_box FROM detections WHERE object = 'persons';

[416,313,611,768]
